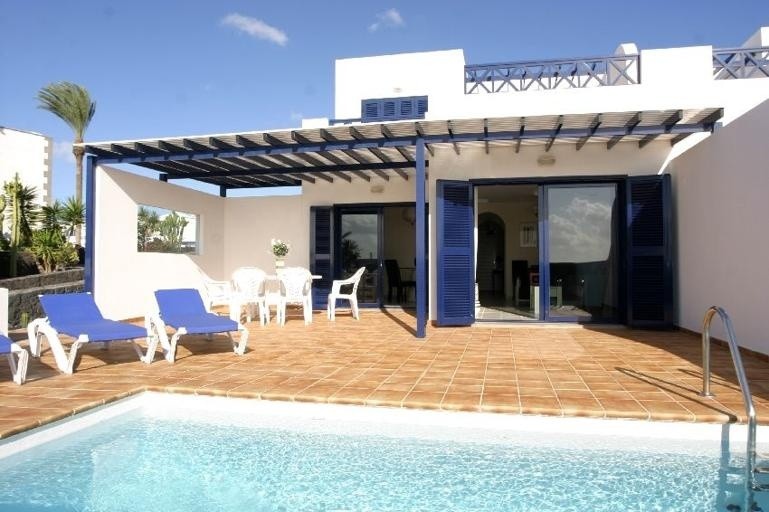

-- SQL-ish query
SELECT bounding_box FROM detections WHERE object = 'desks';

[528,284,567,317]
[400,267,416,305]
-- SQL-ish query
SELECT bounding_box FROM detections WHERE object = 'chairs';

[0,287,29,386]
[197,265,366,327]
[383,259,416,305]
[29,291,154,375]
[145,289,249,364]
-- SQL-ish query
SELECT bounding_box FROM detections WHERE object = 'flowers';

[271,240,289,257]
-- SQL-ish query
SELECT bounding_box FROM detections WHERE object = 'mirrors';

[341,214,378,304]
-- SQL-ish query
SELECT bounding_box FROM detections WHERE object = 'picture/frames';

[519,221,538,248]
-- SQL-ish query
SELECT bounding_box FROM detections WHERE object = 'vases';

[274,258,287,267]
